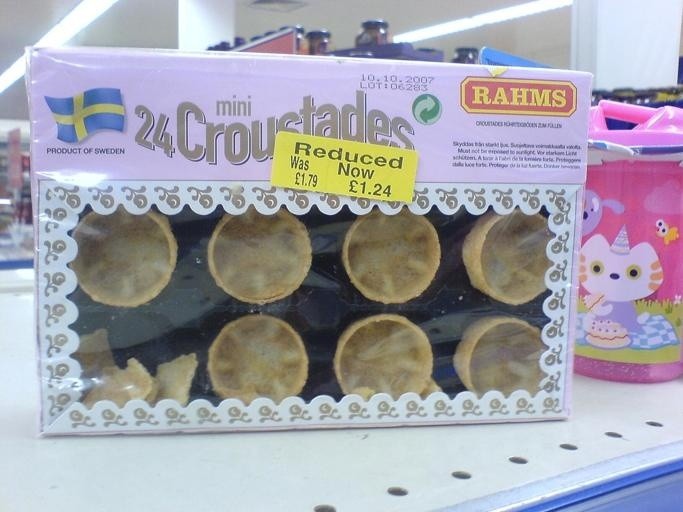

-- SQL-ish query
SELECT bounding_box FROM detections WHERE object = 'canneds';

[279,26,306,54]
[308,29,332,56]
[447,48,479,64]
[354,20,392,48]
